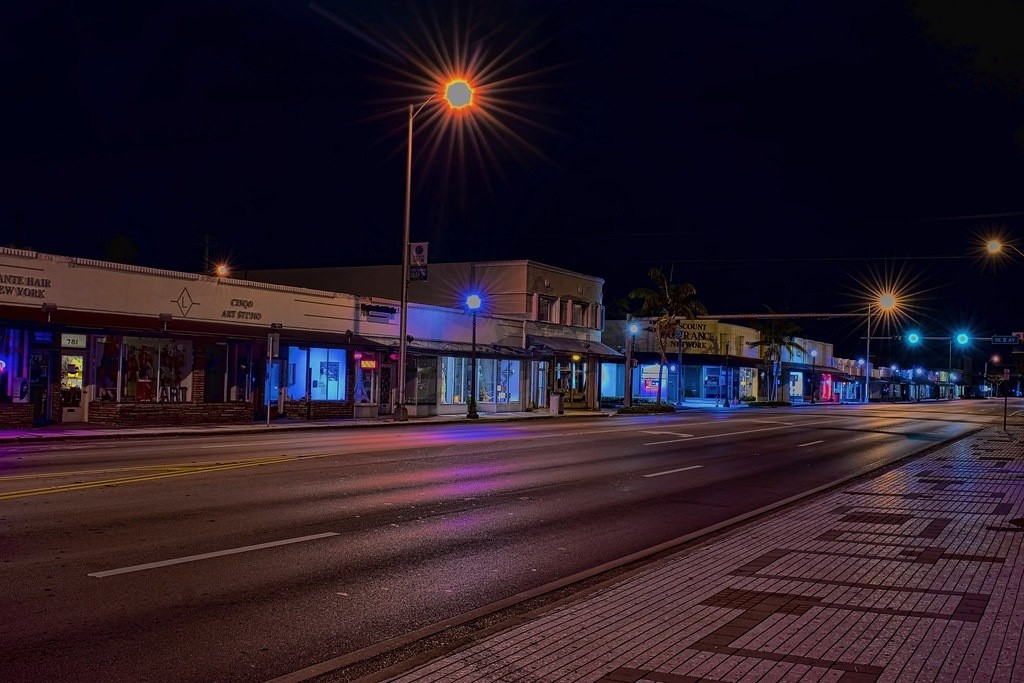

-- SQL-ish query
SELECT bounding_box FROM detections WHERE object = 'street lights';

[984,356,999,377]
[917,369,924,402]
[393,79,484,421]
[865,295,897,406]
[936,372,940,401]
[628,324,643,409]
[948,333,970,400]
[810,350,817,404]
[466,295,481,420]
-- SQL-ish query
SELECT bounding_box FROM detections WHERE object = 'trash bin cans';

[834,393,839,403]
[951,393,953,399]
[550,392,565,415]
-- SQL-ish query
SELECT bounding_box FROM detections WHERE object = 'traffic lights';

[956,332,970,346]
[908,332,921,345]
